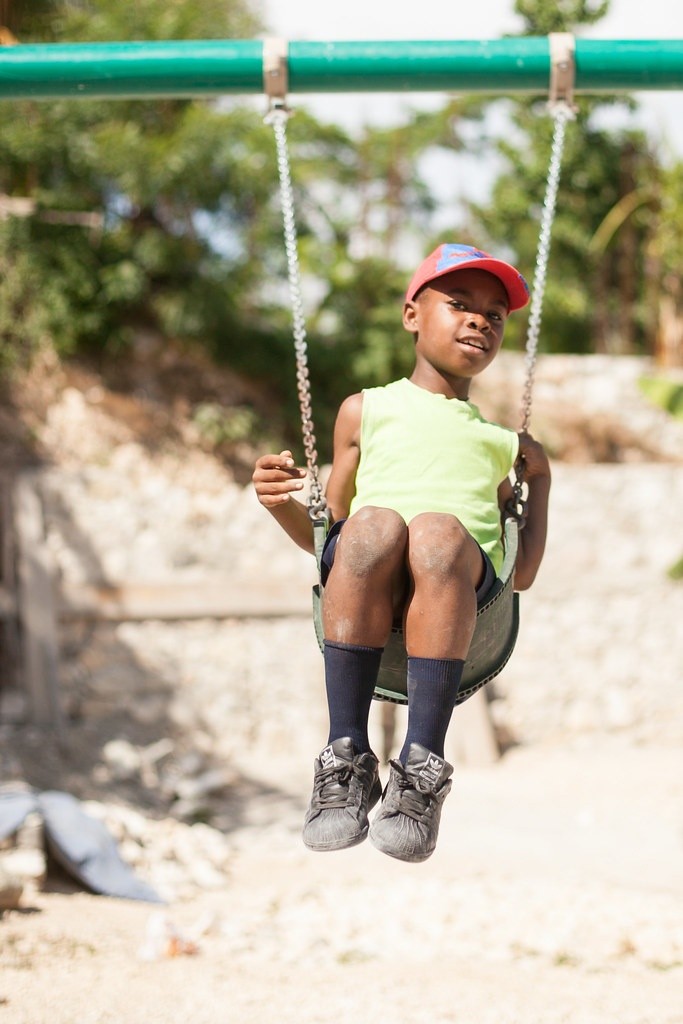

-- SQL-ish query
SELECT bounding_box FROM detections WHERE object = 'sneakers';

[369,741,455,864]
[302,736,382,852]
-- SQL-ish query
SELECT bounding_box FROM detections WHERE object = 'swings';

[256,30,582,715]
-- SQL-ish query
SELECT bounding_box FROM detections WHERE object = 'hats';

[405,244,530,315]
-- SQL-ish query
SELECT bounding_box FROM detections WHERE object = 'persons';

[254,243,555,862]
